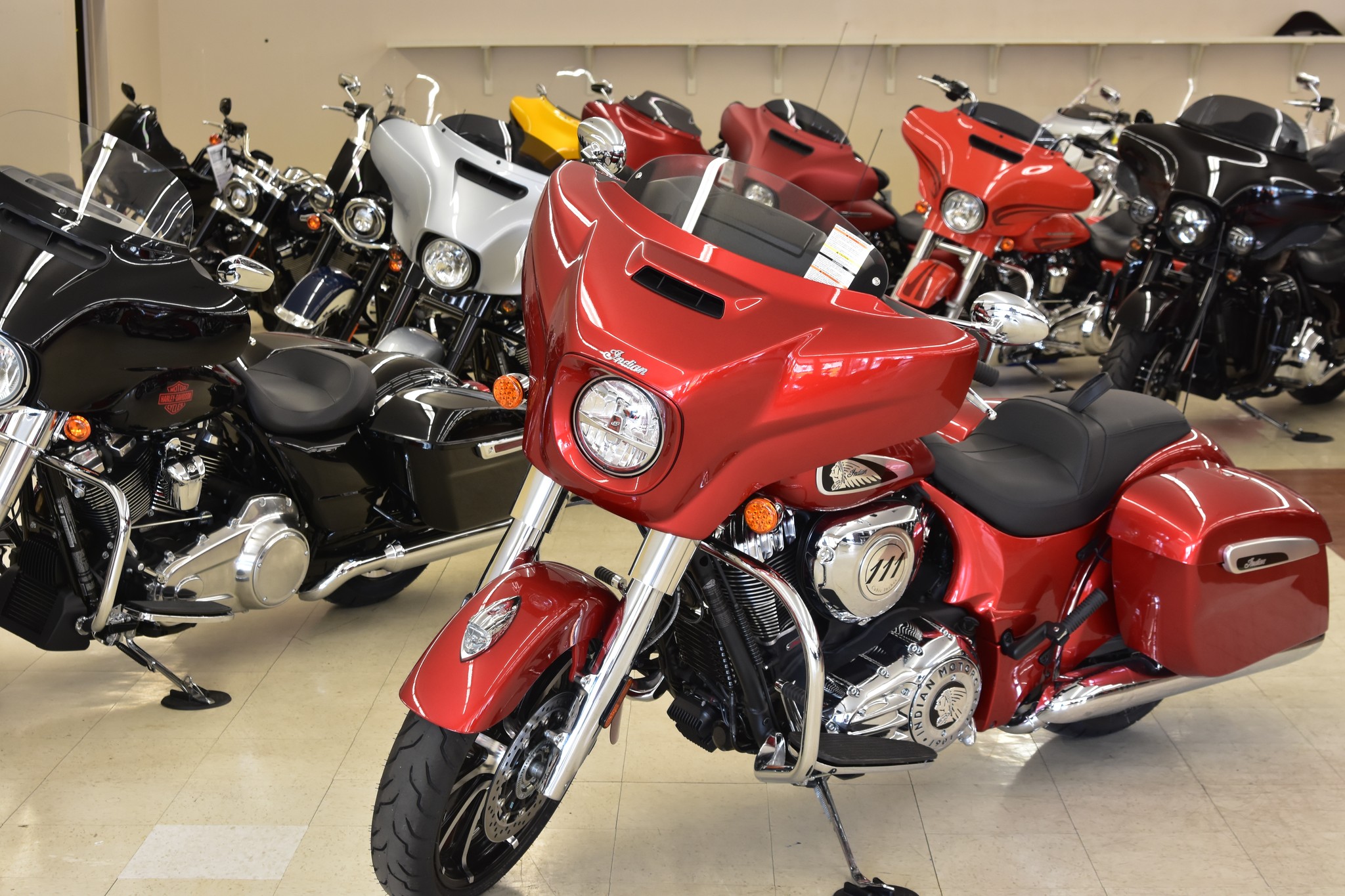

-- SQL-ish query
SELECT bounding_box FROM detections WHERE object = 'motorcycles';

[0,57,1345,712]
[369,124,1337,896]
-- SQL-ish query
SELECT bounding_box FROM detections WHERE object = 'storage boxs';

[1175,95,1307,155]
[367,387,534,533]
[241,333,362,370]
[936,397,1006,444]
[1105,461,1334,678]
